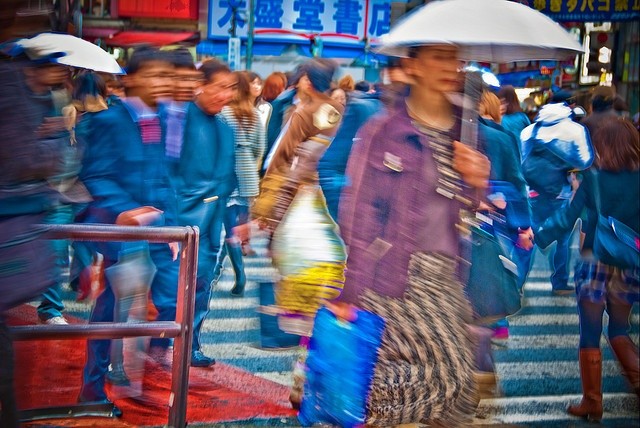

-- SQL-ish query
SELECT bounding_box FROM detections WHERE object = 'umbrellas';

[586,75,587,80]
[374,0,586,93]
[15,33,128,82]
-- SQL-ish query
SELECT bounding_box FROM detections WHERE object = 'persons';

[476,90,521,169]
[0,71,107,338]
[250,60,344,409]
[454,70,532,347]
[77,46,215,416]
[328,45,490,427]
[185,59,269,368]
[521,94,591,295]
[516,118,640,422]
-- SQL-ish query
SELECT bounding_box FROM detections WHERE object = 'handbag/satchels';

[460,226,523,319]
[521,121,575,199]
[590,166,639,269]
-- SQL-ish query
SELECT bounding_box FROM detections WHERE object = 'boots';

[225,240,246,296]
[611,335,640,397]
[568,348,603,421]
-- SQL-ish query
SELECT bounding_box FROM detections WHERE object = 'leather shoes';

[191,350,215,367]
[105,363,130,386]
[78,396,122,416]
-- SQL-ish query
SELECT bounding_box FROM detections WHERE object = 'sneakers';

[38,314,69,325]
